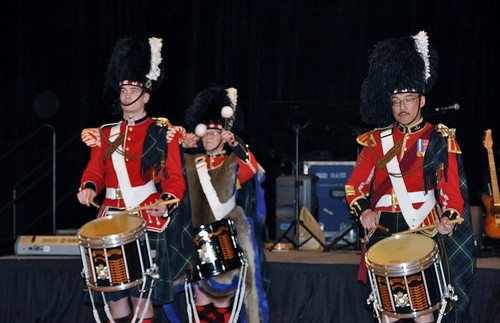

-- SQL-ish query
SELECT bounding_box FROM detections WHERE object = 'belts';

[375,190,435,208]
[106,180,157,199]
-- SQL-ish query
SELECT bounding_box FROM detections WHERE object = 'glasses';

[391,95,419,107]
[205,132,221,136]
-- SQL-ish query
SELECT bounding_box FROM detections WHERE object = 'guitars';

[481,129,500,237]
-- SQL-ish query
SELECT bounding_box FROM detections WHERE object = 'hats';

[202,117,233,131]
[392,68,428,95]
[118,69,153,92]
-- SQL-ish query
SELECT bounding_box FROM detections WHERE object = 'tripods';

[265,100,327,252]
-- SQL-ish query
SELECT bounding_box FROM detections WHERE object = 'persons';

[344,93,464,323]
[178,129,267,323]
[77,85,186,323]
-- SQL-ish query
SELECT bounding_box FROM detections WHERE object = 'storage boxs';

[298,160,357,245]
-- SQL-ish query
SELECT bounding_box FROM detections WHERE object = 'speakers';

[274,174,319,243]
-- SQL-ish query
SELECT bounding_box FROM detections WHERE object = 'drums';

[180,216,244,285]
[364,234,450,319]
[79,215,154,293]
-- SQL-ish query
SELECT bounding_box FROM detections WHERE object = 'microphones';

[436,103,460,111]
